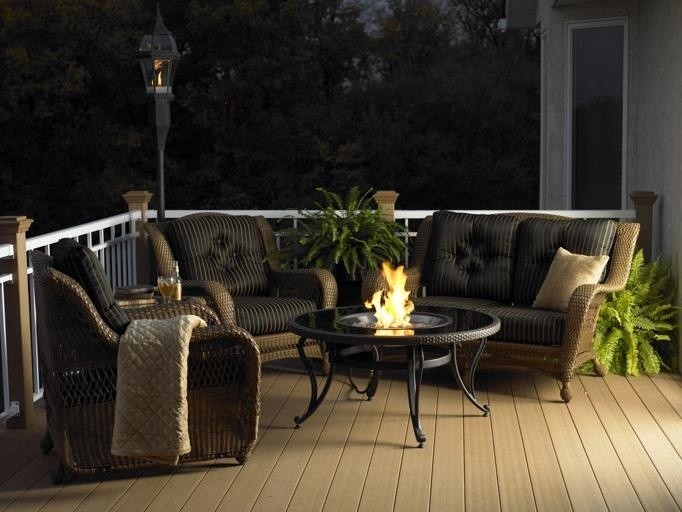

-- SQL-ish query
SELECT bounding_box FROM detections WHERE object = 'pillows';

[532,246,610,312]
[49,239,129,332]
[170,217,267,298]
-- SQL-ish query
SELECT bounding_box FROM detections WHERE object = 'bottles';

[168,260,183,304]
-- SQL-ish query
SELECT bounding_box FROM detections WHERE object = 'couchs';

[364,212,640,402]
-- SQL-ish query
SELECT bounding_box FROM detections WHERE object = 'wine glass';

[156,260,177,307]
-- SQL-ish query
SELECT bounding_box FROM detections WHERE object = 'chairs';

[143,213,339,372]
[28,248,262,485]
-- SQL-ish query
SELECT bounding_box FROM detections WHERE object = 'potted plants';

[261,186,412,308]
